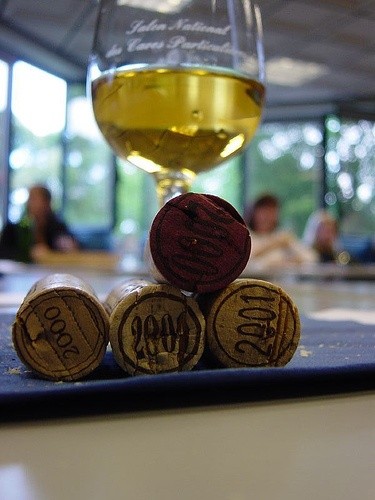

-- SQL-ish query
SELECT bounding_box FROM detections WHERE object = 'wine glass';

[82,0,266,212]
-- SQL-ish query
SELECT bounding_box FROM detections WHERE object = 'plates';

[0,312,375,399]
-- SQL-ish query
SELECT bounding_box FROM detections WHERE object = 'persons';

[243,194,319,264]
[14,184,81,264]
[305,195,342,263]
